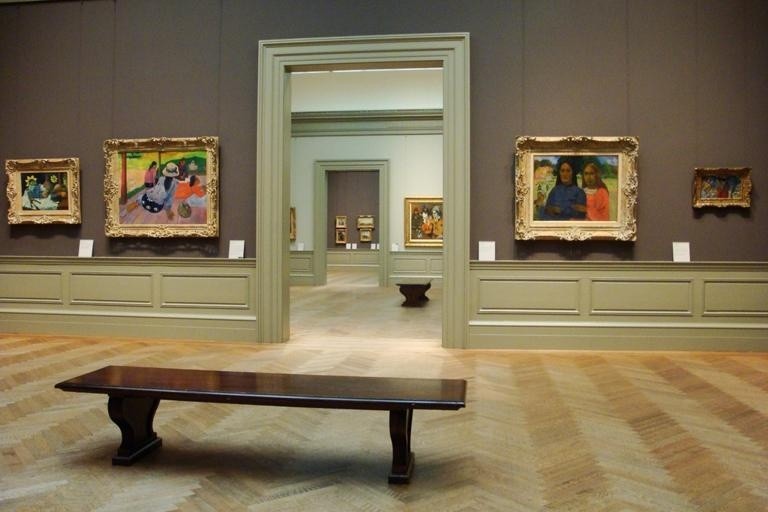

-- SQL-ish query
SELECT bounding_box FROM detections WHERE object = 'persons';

[412,206,442,239]
[126,157,206,220]
[544,159,610,221]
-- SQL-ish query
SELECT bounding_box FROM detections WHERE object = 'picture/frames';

[403,196,444,249]
[692,165,753,211]
[5,157,81,226]
[512,135,640,244]
[334,214,376,244]
[102,135,222,240]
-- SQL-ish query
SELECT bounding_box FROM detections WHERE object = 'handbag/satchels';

[141,192,165,213]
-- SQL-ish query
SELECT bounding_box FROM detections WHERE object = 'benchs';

[394,277,435,308]
[53,363,467,486]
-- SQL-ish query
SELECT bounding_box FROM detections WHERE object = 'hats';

[162,163,180,177]
